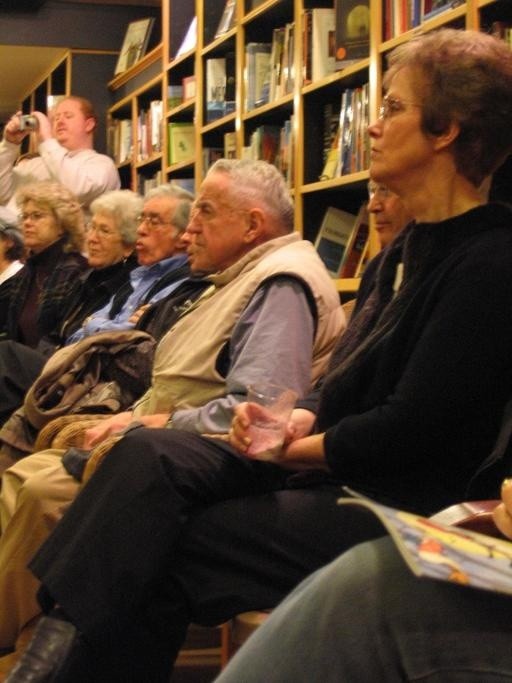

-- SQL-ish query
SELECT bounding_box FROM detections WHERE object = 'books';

[108,1,512,278]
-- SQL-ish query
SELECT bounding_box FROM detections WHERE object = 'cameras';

[19,114,38,130]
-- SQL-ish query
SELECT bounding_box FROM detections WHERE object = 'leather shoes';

[5,616,94,683]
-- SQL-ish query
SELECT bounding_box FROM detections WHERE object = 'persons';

[1,28,511,682]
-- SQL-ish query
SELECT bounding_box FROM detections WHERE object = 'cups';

[245,381,299,463]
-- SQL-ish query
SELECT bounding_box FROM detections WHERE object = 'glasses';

[18,211,53,220]
[87,224,122,239]
[134,217,172,230]
[382,97,423,117]
[368,181,387,201]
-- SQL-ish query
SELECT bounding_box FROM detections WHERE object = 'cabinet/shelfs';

[17,0,512,292]
